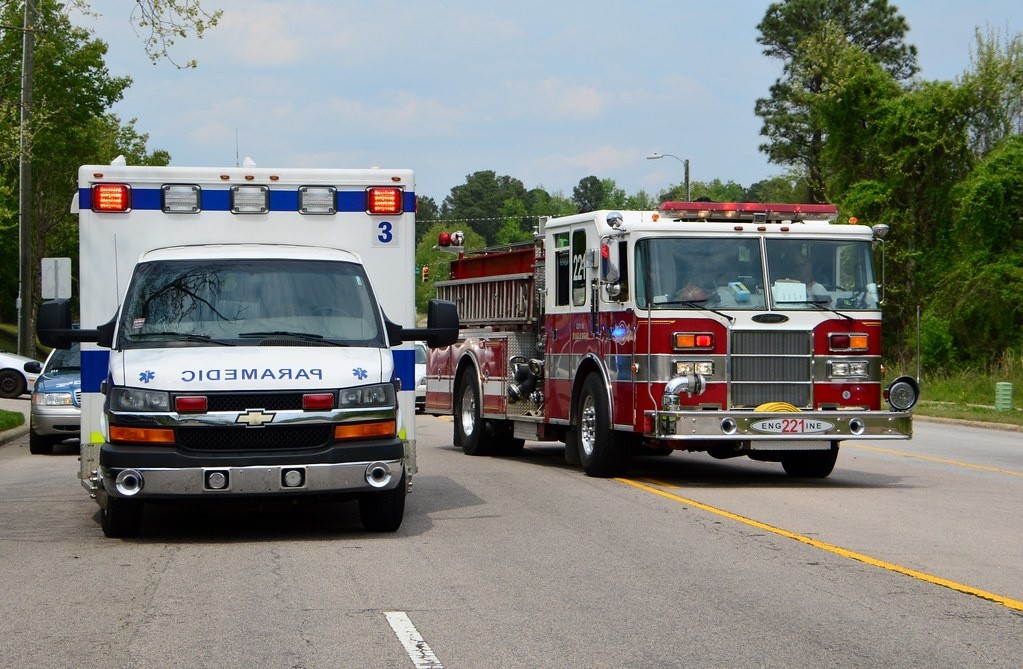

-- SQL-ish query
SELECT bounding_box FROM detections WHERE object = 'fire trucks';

[427,198,919,482]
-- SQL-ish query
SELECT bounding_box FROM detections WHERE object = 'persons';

[788,254,829,291]
[306,275,356,319]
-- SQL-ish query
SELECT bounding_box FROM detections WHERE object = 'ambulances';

[38,163,417,538]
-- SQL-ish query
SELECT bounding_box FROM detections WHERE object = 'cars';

[0,349,44,398]
[24,341,81,456]
[414,340,432,411]
